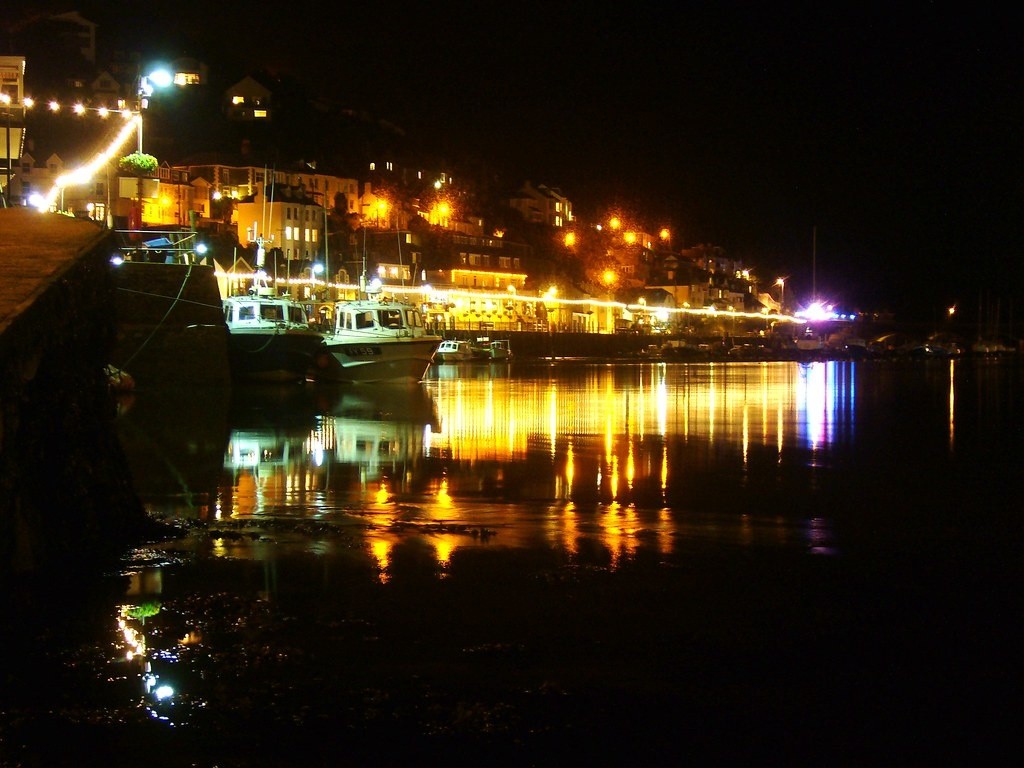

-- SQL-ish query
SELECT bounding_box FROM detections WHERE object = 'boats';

[640,333,1019,364]
[325,384,442,469]
[486,337,514,361]
[317,257,443,383]
[220,245,325,359]
[434,340,488,361]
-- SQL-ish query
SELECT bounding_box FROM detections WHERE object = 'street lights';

[133,70,171,261]
[509,284,517,316]
[637,297,648,322]
[775,276,785,300]
